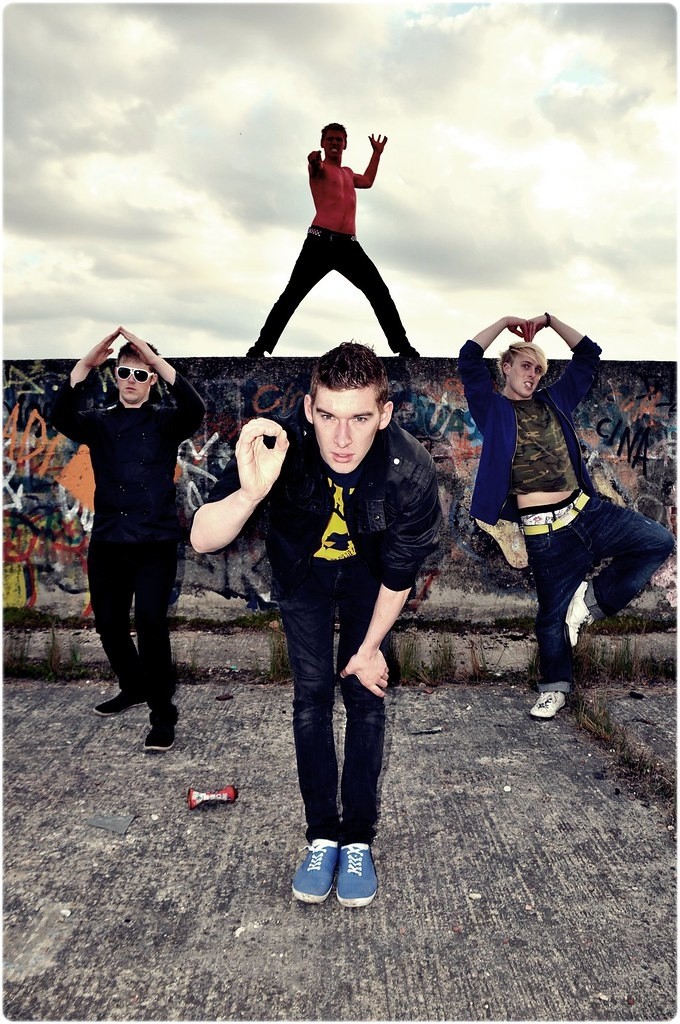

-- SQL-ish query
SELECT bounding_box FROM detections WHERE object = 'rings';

[342,669,348,677]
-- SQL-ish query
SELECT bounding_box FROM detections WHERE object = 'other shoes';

[398,346,420,357]
[142,725,176,751]
[246,346,264,360]
[94,691,148,716]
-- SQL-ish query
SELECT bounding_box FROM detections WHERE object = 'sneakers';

[566,580,594,647]
[530,691,566,720]
[292,840,338,902]
[335,843,378,907]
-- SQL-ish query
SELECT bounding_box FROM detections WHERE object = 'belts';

[522,492,591,535]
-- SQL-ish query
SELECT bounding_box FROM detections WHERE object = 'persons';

[246,123,421,358]
[456,313,675,720]
[185,341,442,909]
[48,326,205,752]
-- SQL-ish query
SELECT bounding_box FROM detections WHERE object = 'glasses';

[116,366,155,383]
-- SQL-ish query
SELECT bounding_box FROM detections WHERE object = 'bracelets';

[544,312,550,328]
